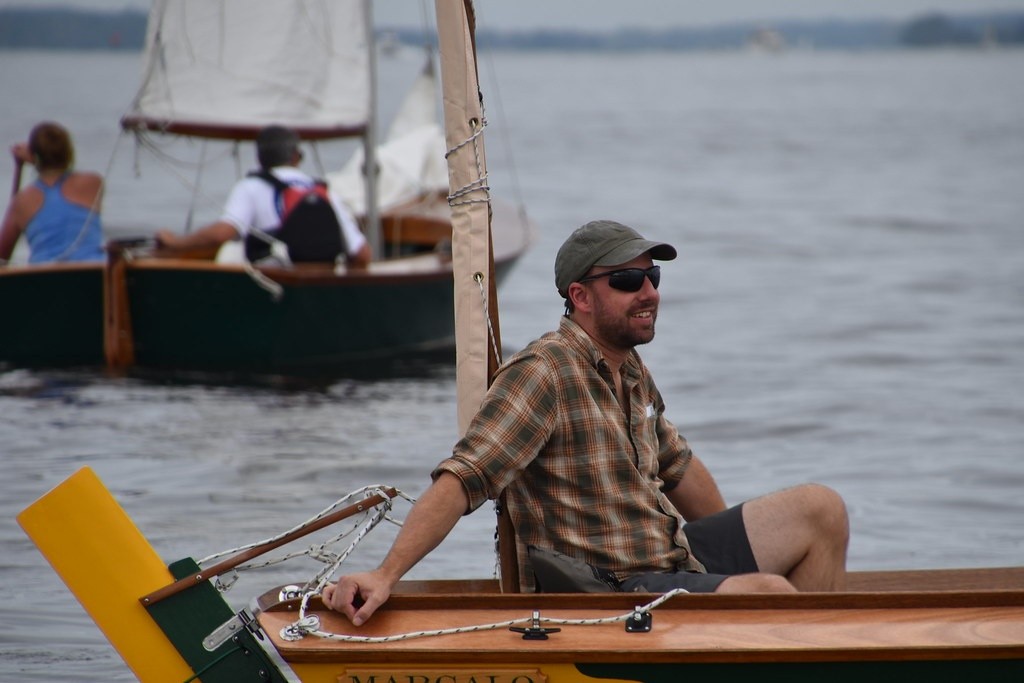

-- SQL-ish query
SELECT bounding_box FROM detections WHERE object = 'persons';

[0,120,106,266]
[321,220,851,626]
[154,125,374,265]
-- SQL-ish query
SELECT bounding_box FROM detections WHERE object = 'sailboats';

[12,2,1024,683]
[0,2,542,391]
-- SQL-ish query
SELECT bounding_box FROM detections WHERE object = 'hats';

[555,219,677,298]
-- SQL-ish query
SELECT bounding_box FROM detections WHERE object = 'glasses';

[580,266,660,292]
